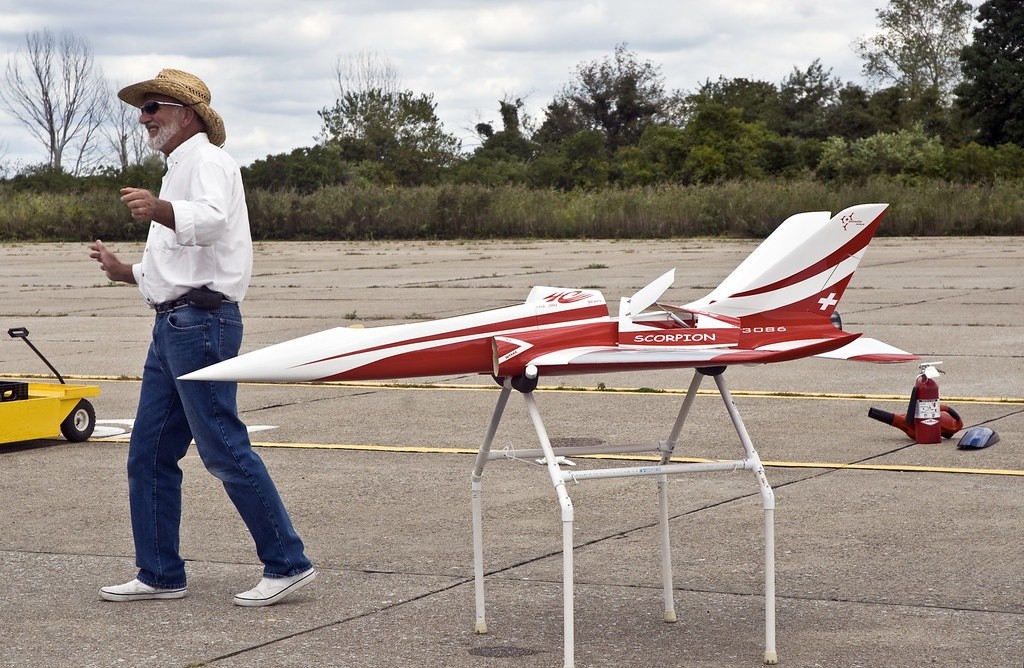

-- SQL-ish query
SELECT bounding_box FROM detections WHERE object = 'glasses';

[141,101,183,114]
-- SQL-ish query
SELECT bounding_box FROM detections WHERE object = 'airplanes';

[175,205,920,385]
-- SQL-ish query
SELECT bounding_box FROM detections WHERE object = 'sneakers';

[233,567,315,606]
[99,578,187,601]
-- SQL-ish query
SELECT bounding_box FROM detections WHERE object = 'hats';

[117,68,226,147]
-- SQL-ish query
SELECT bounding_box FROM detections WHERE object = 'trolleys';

[0,326,102,447]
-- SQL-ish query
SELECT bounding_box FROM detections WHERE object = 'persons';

[90,68,316,607]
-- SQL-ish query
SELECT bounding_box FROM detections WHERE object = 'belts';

[155,295,236,313]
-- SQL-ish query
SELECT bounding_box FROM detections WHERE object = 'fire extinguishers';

[905,362,946,444]
